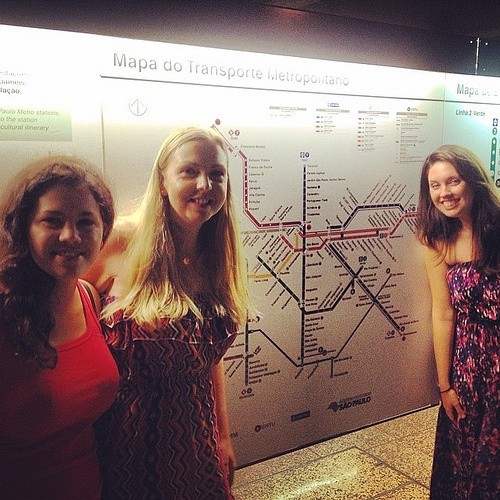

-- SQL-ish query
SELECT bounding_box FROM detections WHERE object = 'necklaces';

[180,254,193,264]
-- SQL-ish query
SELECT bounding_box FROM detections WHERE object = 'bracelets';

[440,386,453,393]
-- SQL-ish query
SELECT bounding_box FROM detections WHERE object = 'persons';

[0,154,120,500]
[76,128,258,500]
[415,145,500,500]
